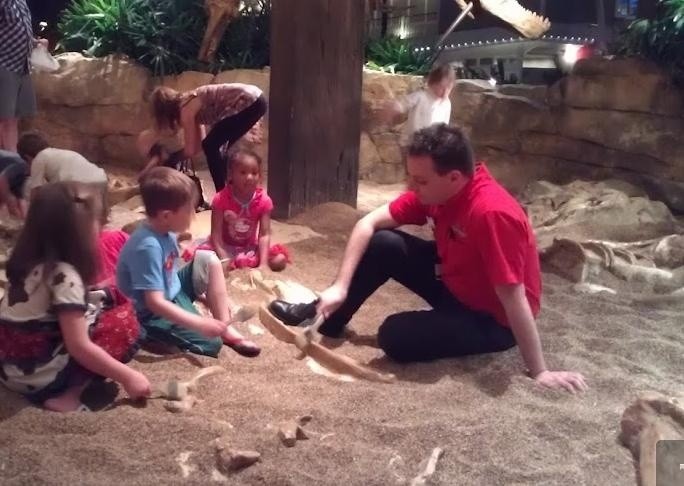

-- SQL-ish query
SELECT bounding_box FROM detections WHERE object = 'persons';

[266,122,593,397]
[117,168,261,358]
[195,148,288,279]
[148,82,268,194]
[0,1,49,152]
[386,61,457,177]
[1,131,152,415]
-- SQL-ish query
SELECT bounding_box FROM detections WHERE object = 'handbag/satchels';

[180,172,204,208]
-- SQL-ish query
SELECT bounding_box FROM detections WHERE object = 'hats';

[137,129,162,155]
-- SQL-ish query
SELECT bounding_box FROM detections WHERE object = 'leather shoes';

[270,298,343,338]
[224,336,261,357]
